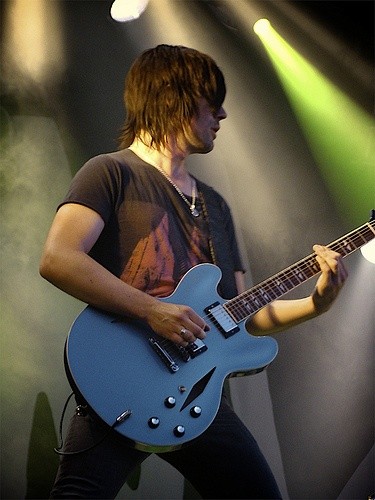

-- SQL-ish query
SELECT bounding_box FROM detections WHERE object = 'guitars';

[64,208,375,453]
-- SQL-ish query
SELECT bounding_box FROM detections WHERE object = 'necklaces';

[148,157,200,219]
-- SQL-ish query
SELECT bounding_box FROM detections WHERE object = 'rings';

[179,327,187,337]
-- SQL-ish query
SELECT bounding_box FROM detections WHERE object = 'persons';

[38,45,351,500]
